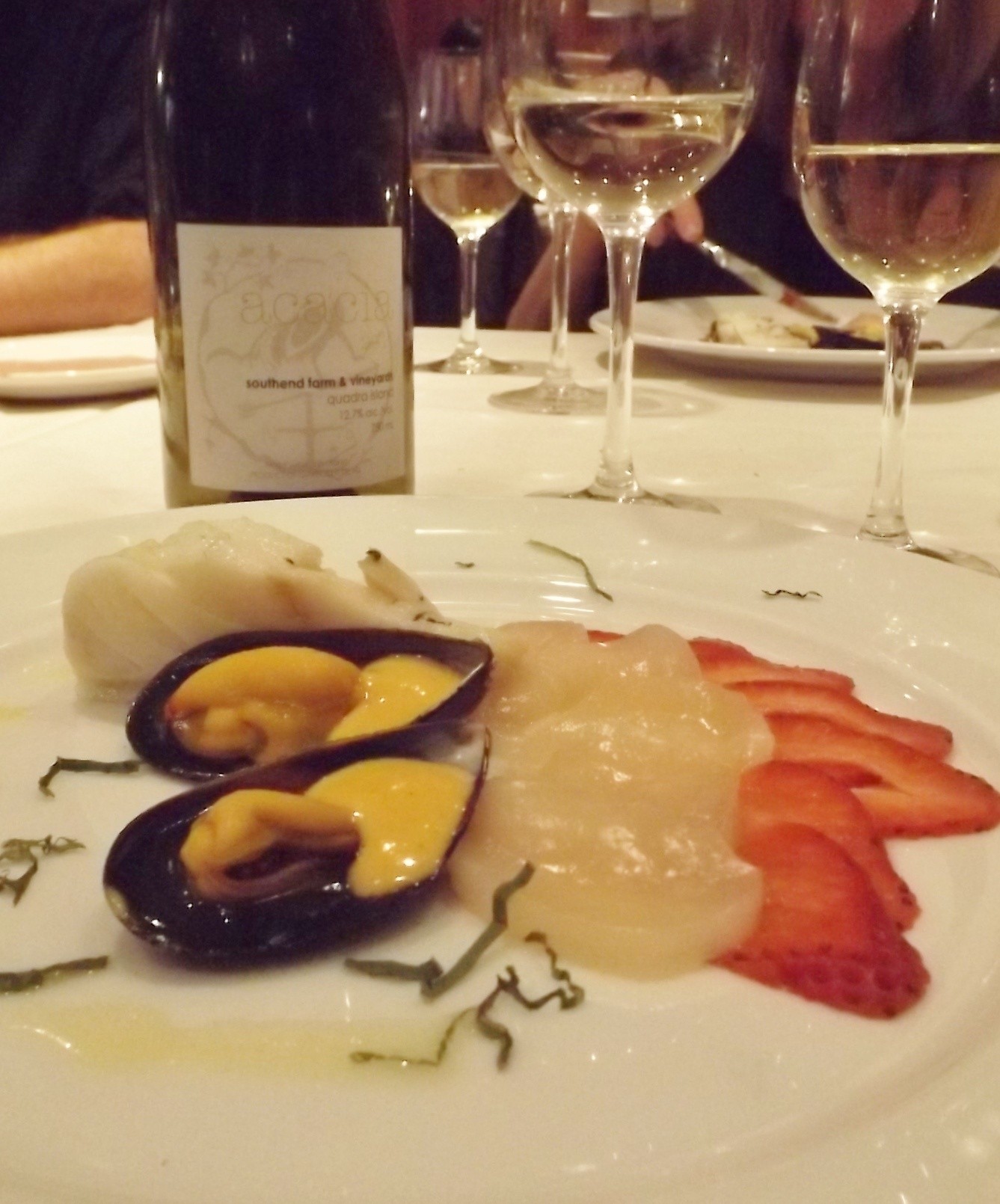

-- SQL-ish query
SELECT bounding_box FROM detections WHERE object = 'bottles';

[144,1,415,508]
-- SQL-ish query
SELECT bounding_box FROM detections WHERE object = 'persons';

[0,0,868,335]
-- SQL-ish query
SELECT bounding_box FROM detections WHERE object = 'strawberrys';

[580,630,1000,1016]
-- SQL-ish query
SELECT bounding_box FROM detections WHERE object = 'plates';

[587,297,1000,383]
[0,325,157,400]
[0,495,1000,1203]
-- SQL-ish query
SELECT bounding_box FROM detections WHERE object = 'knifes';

[686,232,836,322]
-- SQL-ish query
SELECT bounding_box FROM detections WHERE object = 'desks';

[0,325,999,572]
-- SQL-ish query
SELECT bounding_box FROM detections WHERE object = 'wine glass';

[496,0,769,515]
[791,0,1000,577]
[413,56,524,373]
[479,0,658,415]
